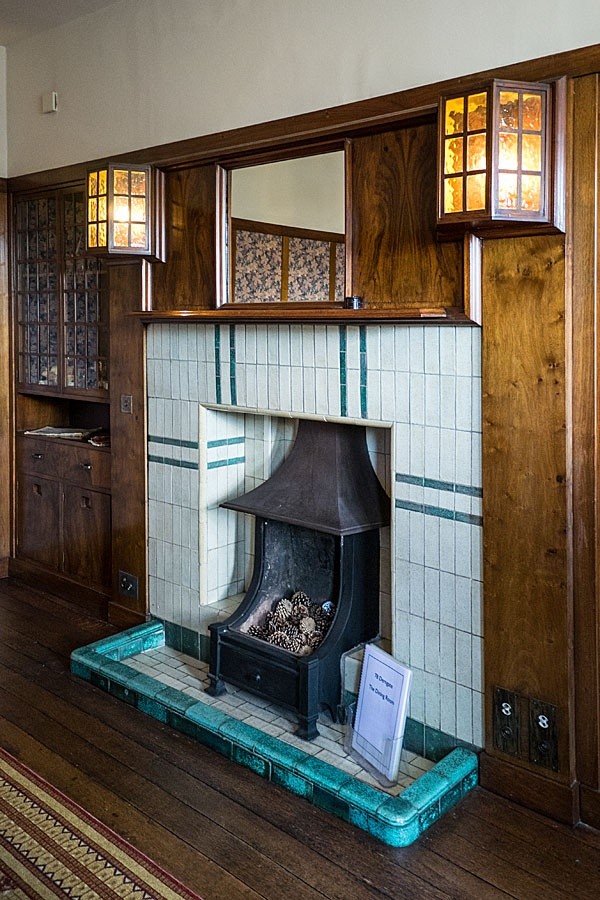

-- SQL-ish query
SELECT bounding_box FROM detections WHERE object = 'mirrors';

[214,137,354,309]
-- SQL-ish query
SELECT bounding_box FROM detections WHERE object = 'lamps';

[83,162,167,267]
[431,75,569,241]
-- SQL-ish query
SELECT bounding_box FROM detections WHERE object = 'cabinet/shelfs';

[12,179,111,405]
[15,431,111,622]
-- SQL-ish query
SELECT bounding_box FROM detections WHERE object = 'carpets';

[0,746,204,900]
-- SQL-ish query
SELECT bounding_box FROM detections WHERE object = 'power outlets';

[527,696,560,774]
[118,569,138,601]
[493,684,523,760]
[121,394,133,415]
